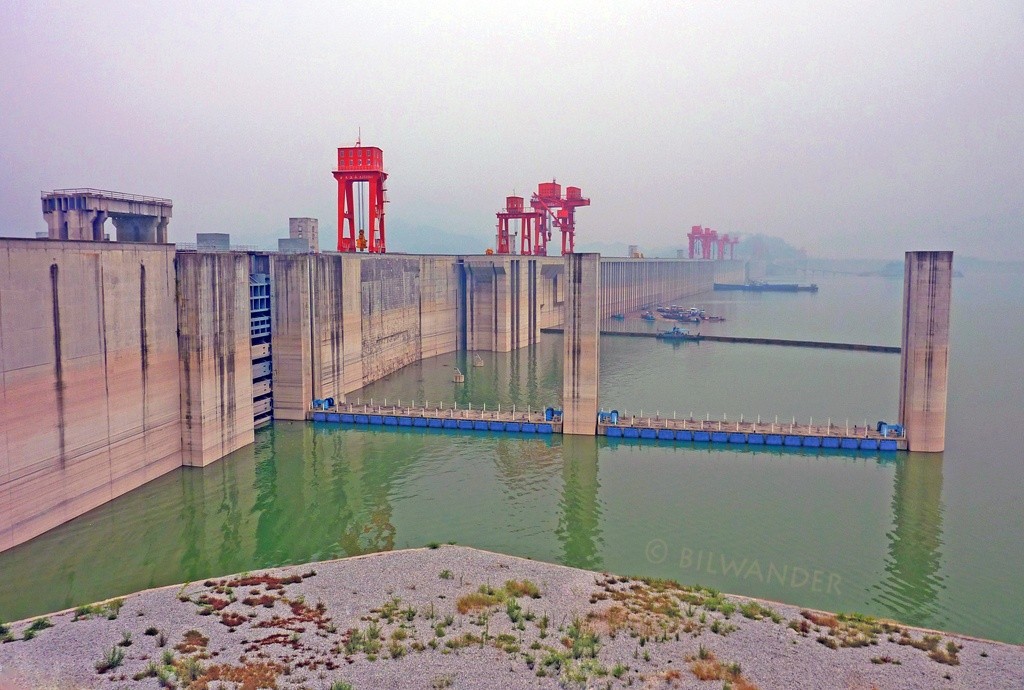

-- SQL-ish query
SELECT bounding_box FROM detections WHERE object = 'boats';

[641,310,656,321]
[655,323,700,340]
[656,303,726,322]
[611,313,624,318]
[713,281,818,293]
